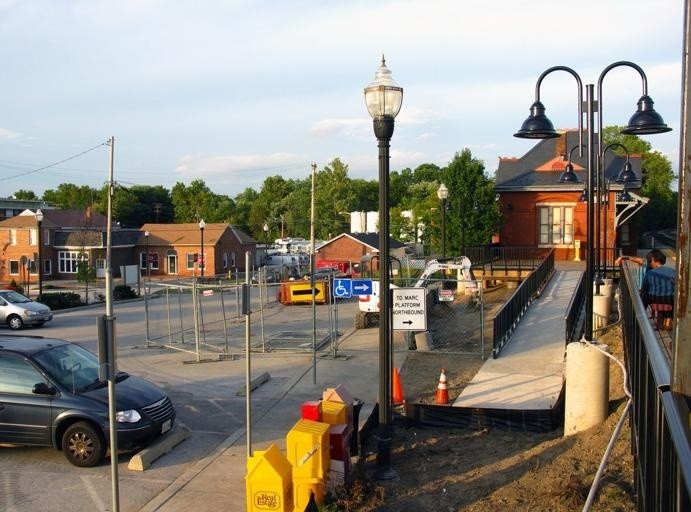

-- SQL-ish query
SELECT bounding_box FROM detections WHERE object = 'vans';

[0,330,176,473]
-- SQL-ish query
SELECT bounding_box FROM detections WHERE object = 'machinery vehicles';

[350,255,482,332]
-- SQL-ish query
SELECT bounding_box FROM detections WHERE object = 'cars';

[251,263,290,288]
[0,287,57,332]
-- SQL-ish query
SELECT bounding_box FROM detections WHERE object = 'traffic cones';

[391,366,406,408]
[434,367,451,405]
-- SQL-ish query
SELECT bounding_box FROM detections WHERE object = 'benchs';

[648,303,672,330]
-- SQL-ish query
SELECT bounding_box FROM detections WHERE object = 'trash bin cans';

[317,397,364,461]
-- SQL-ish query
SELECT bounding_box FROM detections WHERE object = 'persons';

[640,249,676,329]
[615,249,661,268]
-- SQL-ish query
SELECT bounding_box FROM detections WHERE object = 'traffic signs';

[388,288,430,332]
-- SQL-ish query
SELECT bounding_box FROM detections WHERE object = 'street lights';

[144,229,152,296]
[308,160,319,388]
[355,49,411,469]
[198,217,206,283]
[34,206,45,300]
[263,223,269,264]
[337,211,352,233]
[437,182,449,258]
